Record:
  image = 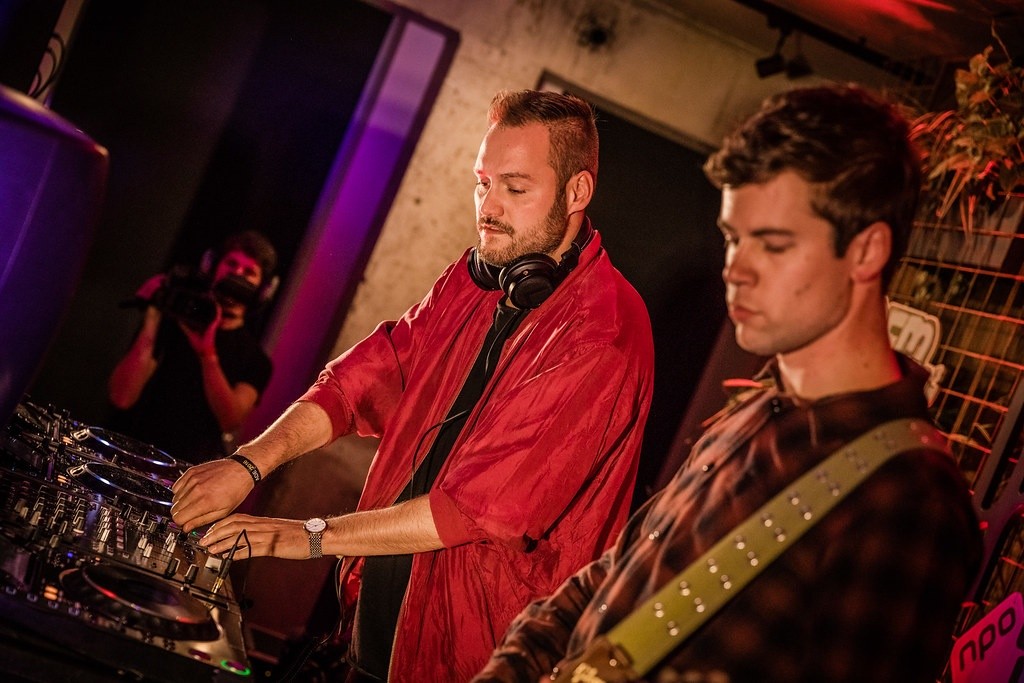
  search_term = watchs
[302,515,327,559]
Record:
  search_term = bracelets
[227,454,262,488]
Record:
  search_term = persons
[170,89,655,683]
[472,86,982,683]
[107,226,279,466]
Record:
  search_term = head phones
[198,249,279,303]
[465,214,594,312]
